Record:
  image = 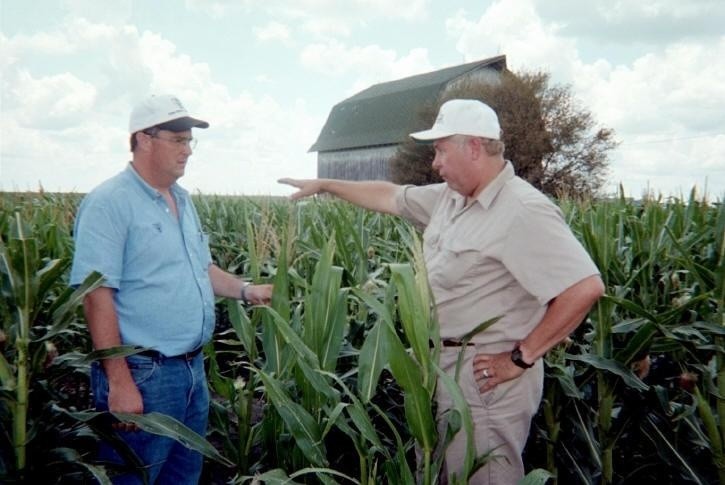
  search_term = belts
[136,345,205,363]
[424,338,476,347]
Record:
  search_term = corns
[680,371,723,453]
[0,352,16,386]
[552,336,569,374]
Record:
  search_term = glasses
[143,133,198,151]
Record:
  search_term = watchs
[510,344,535,369]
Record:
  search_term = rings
[483,369,489,378]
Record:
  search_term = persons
[67,92,275,485]
[277,98,606,485]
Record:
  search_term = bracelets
[241,282,253,305]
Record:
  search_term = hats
[128,93,209,136]
[408,98,501,144]
